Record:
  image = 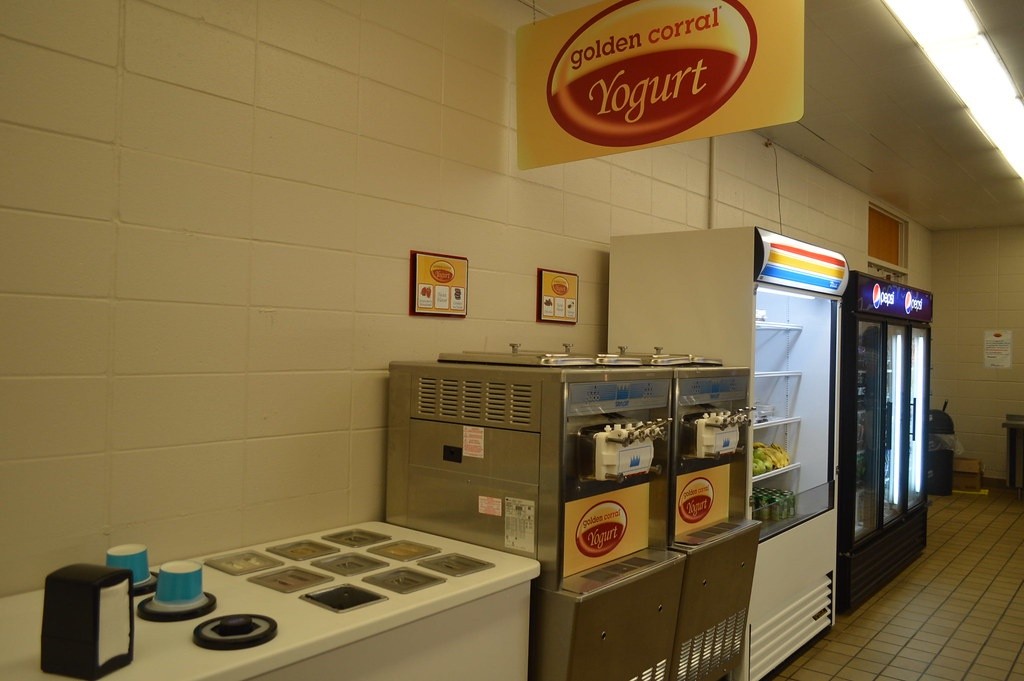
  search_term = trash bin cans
[927,409,955,496]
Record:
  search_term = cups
[153,560,205,607]
[105,544,152,586]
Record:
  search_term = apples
[753,451,772,474]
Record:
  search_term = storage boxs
[952,457,983,493]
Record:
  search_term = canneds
[753,487,794,521]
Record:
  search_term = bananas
[754,442,789,468]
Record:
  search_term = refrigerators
[841,271,933,615]
[604,225,840,681]
